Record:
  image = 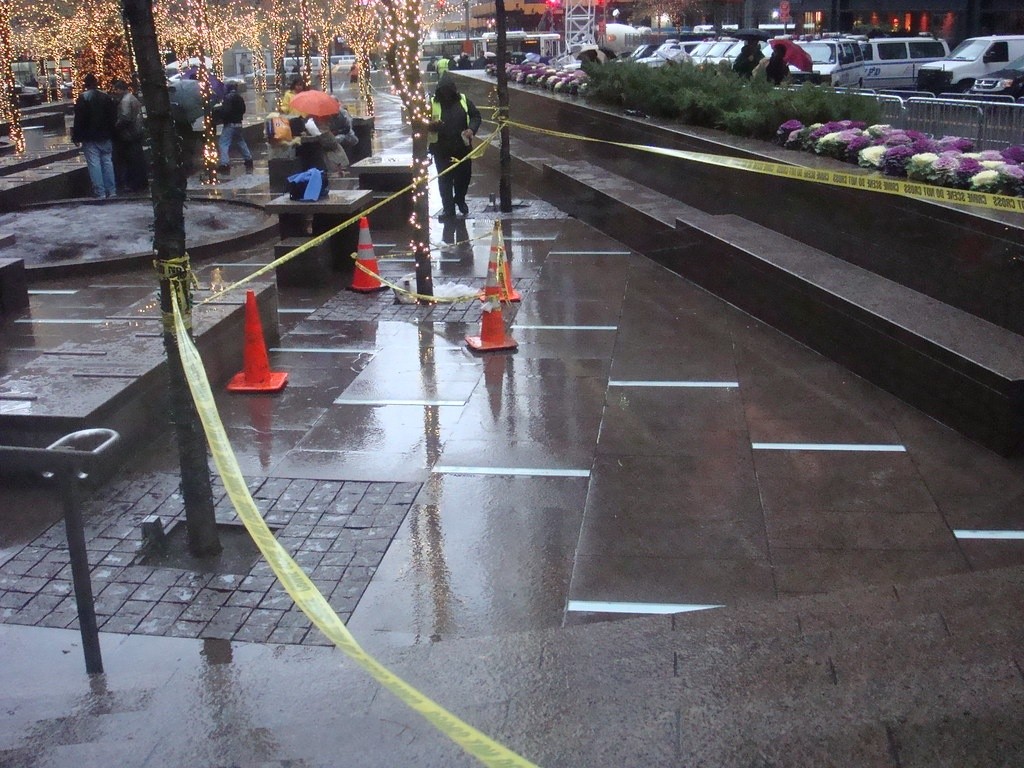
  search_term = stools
[371,192,406,229]
[274,237,332,286]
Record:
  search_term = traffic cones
[226,288,288,392]
[345,217,389,293]
[463,266,519,351]
[478,220,522,304]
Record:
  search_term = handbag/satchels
[267,116,293,142]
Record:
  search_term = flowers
[485,61,590,98]
[776,117,1024,198]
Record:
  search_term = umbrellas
[729,27,772,43]
[512,51,525,55]
[484,51,495,57]
[180,66,225,106]
[168,78,217,122]
[290,89,340,116]
[768,37,813,73]
[525,53,541,63]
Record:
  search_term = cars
[318,64,354,82]
[0,79,41,109]
[243,68,298,87]
[161,71,250,99]
[627,31,1024,99]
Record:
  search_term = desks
[265,190,373,272]
[350,156,414,213]
[351,116,375,157]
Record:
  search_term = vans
[330,54,377,72]
[282,56,334,85]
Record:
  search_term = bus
[416,31,562,69]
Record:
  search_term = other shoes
[96,192,106,199]
[438,207,455,220]
[453,195,468,213]
[244,159,254,174]
[107,191,117,197]
[217,165,230,175]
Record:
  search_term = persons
[764,43,790,86]
[350,58,359,83]
[72,73,117,199]
[214,78,253,173]
[261,79,358,159]
[733,39,765,79]
[426,51,487,79]
[425,71,482,220]
[113,76,146,191]
[506,55,523,64]
